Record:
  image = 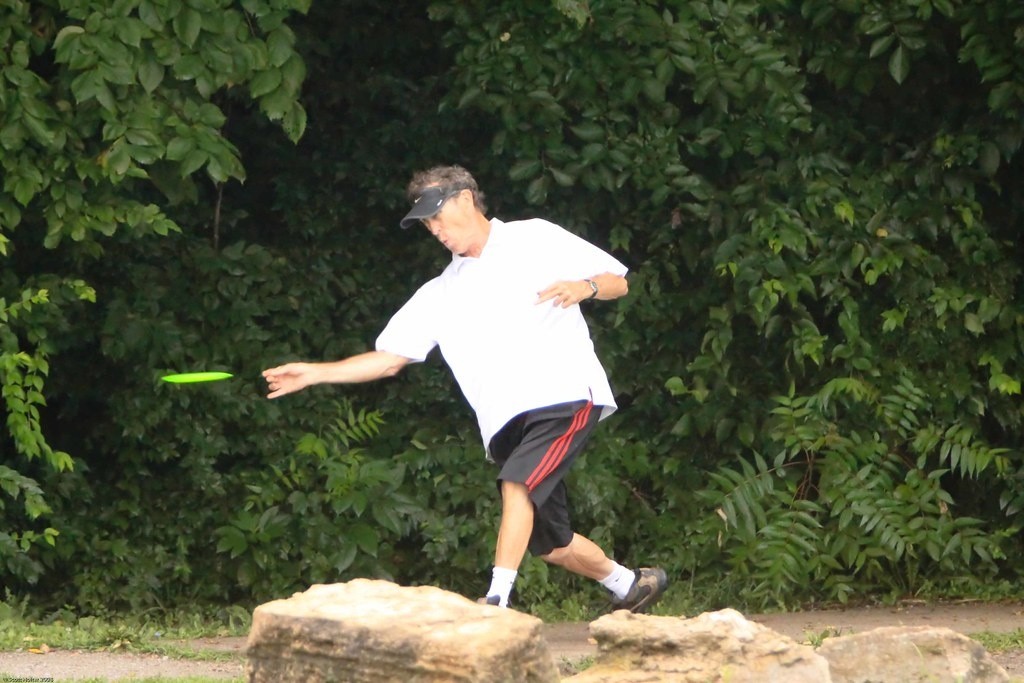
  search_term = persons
[262,166,667,613]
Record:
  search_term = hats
[400,183,470,229]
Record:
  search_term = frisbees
[159,372,234,383]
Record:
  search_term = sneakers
[478,595,511,609]
[611,567,667,614]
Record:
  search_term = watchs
[584,278,598,299]
